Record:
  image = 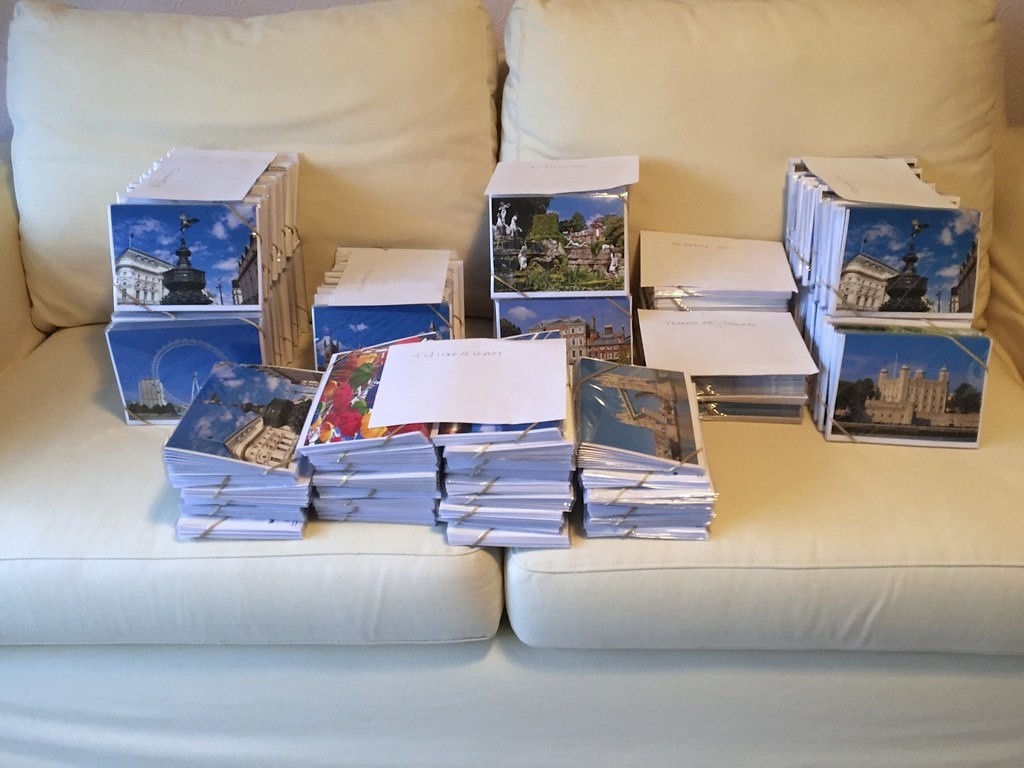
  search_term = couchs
[1,0,1024,767]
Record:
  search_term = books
[633,156,993,448]
[490,192,633,364]
[105,153,309,427]
[311,247,465,375]
[163,330,720,550]
